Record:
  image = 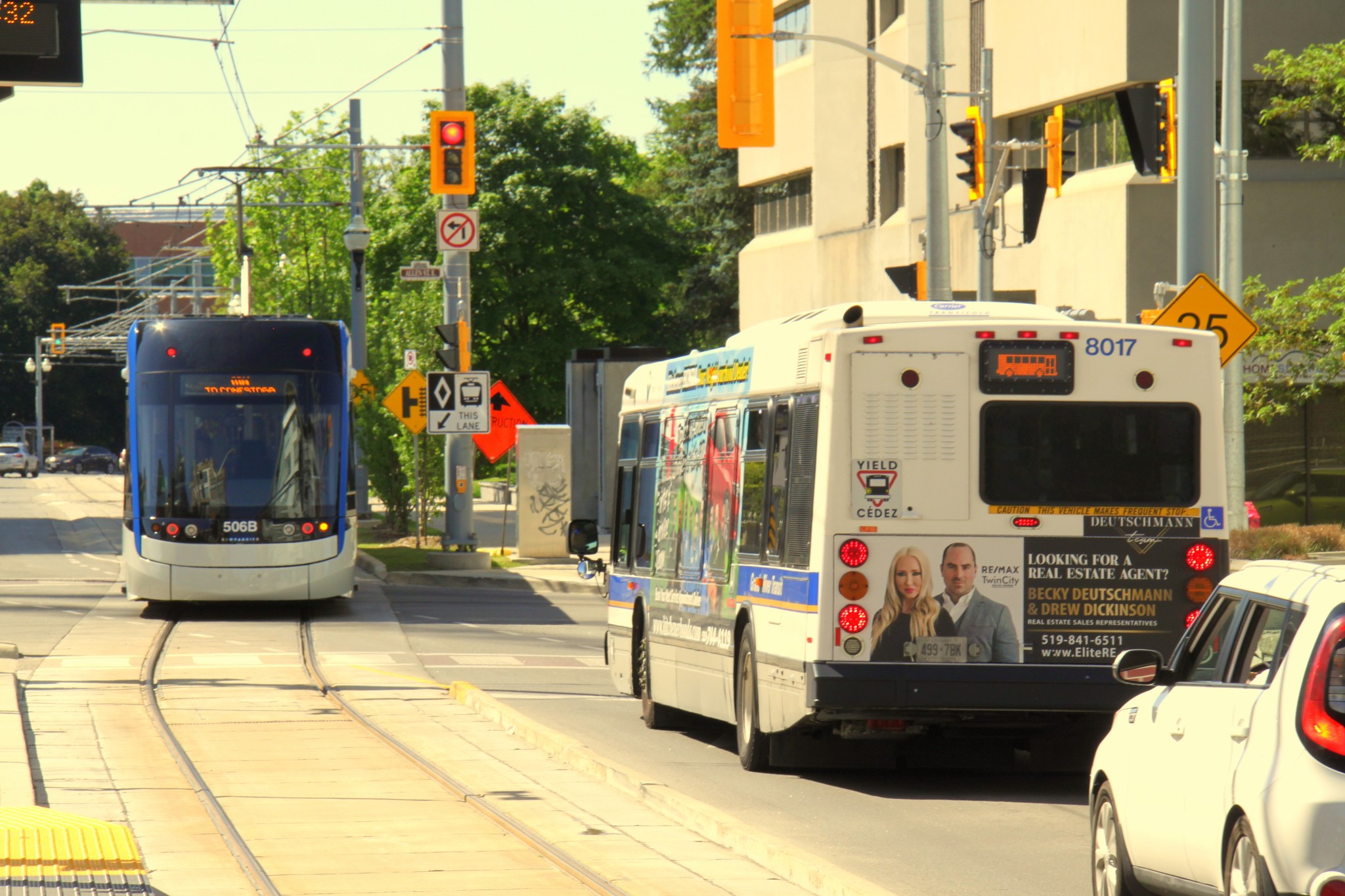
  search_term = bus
[119,319,357,609]
[568,302,1233,775]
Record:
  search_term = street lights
[26,358,53,465]
[119,366,130,476]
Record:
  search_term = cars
[0,441,40,479]
[44,444,118,475]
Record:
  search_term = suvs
[1082,552,1345,896]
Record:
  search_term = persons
[869,546,957,662]
[933,543,1019,663]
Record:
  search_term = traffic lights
[950,106,988,202]
[430,110,477,196]
[0,0,84,86]
[884,263,927,301]
[1046,104,1081,199]
[1115,79,1178,185]
[49,323,66,355]
